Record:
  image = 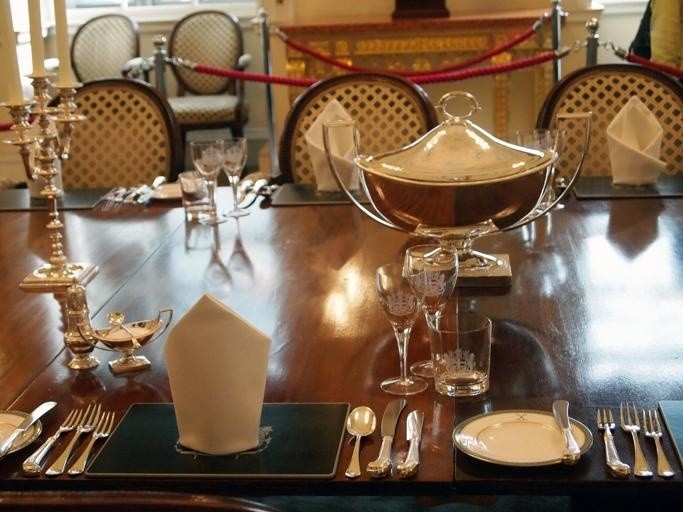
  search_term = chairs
[278,72,438,185]
[65,11,142,87]
[166,9,251,181]
[533,63,683,180]
[42,77,185,184]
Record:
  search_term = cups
[180,168,210,222]
[431,308,494,396]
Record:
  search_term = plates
[452,407,593,470]
[0,406,42,455]
[145,184,185,203]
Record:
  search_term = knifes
[366,397,408,479]
[0,400,58,458]
[396,410,423,477]
[551,397,581,463]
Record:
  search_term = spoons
[345,404,376,480]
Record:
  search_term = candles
[51,1,75,85]
[0,0,23,103]
[26,0,48,79]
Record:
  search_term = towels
[162,292,274,456]
[303,94,359,193]
[604,91,666,191]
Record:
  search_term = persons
[629,0,683,81]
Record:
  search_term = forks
[97,182,143,214]
[642,404,675,479]
[617,399,653,478]
[20,403,115,482]
[593,403,630,477]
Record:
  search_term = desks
[0,179,682,512]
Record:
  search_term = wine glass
[403,245,458,379]
[372,263,433,394]
[189,133,254,225]
[511,126,567,222]
